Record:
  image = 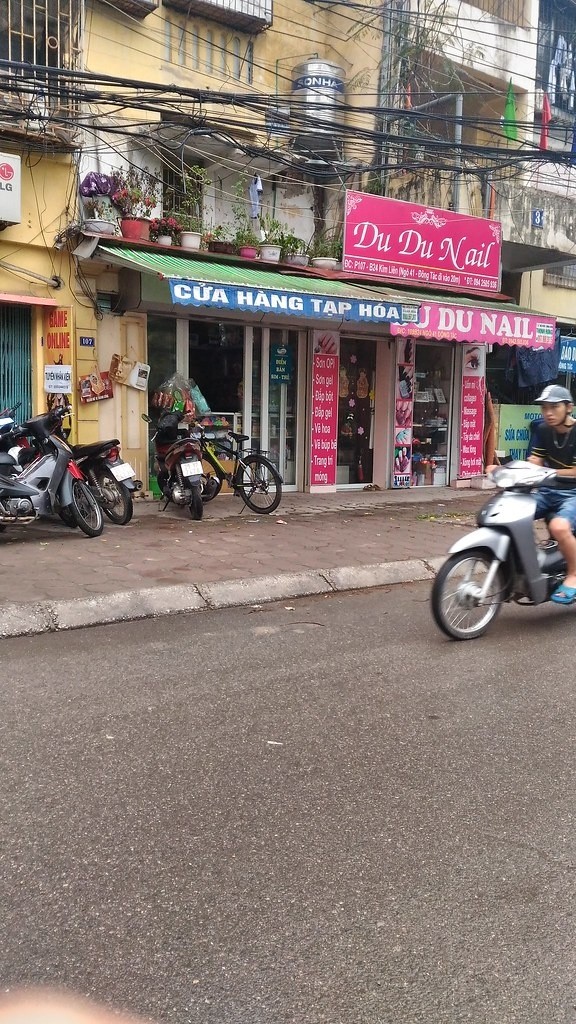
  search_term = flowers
[112,188,158,220]
[149,216,183,236]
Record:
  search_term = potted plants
[83,200,115,234]
[180,165,342,271]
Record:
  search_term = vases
[118,217,153,241]
[158,235,172,246]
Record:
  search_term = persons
[396,401,413,426]
[395,428,411,444]
[394,447,410,473]
[317,332,338,354]
[399,339,413,363]
[397,365,414,399]
[485,385,576,602]
[465,346,485,375]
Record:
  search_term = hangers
[248,166,260,187]
[560,327,576,343]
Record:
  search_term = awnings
[96,243,423,325]
[346,279,558,351]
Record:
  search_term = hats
[534,385,574,403]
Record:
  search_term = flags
[539,94,552,150]
[502,80,517,143]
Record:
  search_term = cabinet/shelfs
[411,371,449,485]
[148,412,297,492]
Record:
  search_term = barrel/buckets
[149,476,162,498]
[291,58,346,157]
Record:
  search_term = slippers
[550,584,576,604]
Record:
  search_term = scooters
[429,459,575,641]
[139,410,204,521]
[0,401,143,537]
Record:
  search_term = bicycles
[189,415,283,514]
